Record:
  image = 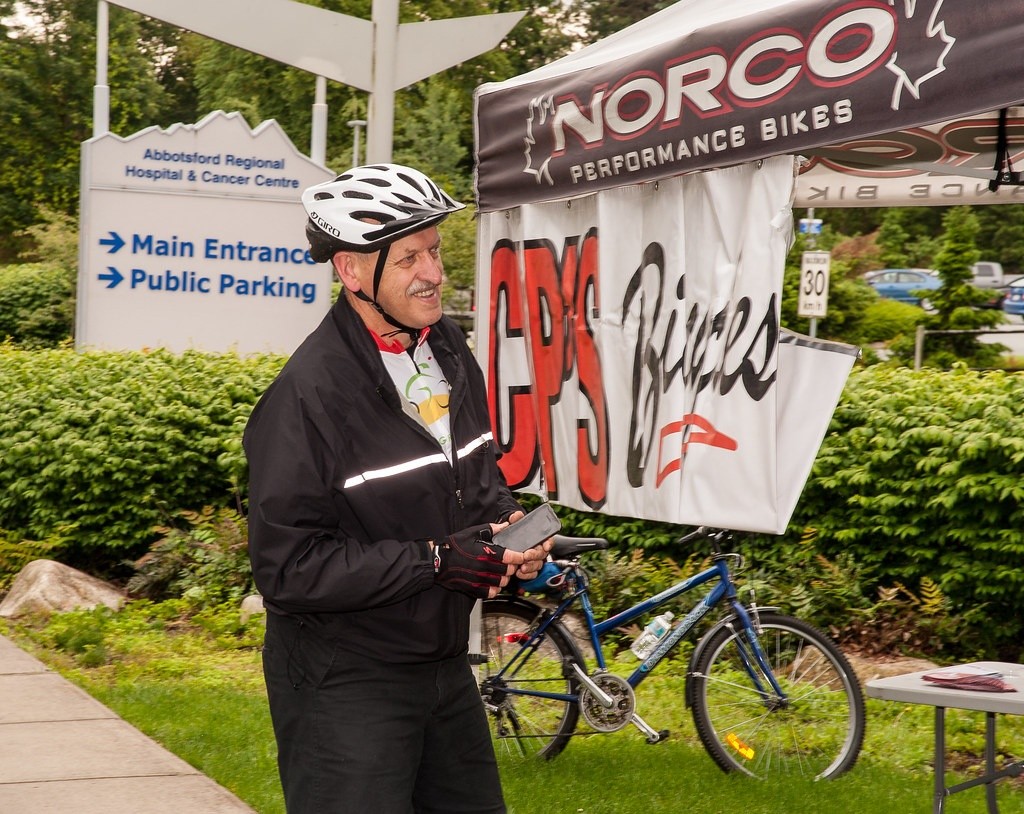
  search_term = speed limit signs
[800,248,832,319]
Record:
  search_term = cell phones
[492,503,562,553]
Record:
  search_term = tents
[469,0,1024,665]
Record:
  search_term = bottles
[629,610,675,661]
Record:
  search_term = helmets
[301,163,467,264]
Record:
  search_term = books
[920,664,1000,683]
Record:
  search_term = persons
[242,161,554,814]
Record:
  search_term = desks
[865,661,1024,814]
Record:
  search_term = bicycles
[472,516,868,794]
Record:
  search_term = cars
[856,267,946,312]
[969,263,1024,315]
